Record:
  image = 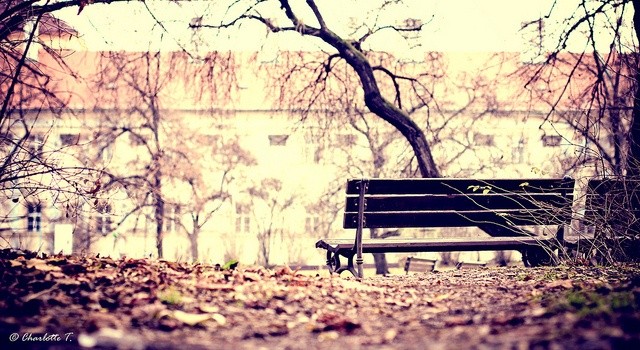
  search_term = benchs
[561,179,640,263]
[314,176,574,279]
